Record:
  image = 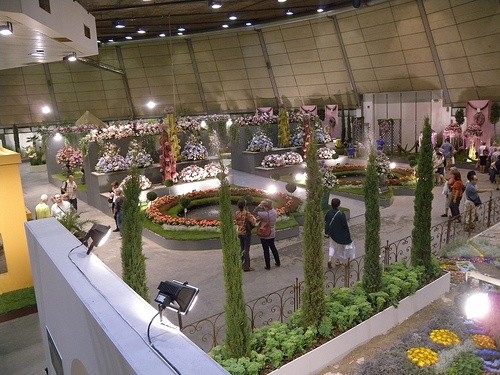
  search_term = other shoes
[328,262,332,268]
[113,228,120,232]
[441,214,448,217]
[264,266,270,271]
[275,262,280,266]
[244,267,255,272]
[335,261,343,266]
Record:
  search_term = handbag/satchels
[256,210,272,237]
[344,242,355,260]
[442,181,450,196]
[61,180,67,194]
[474,196,482,206]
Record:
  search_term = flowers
[24,106,485,244]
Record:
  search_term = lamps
[81,223,111,254]
[155,279,200,316]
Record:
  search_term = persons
[433,137,454,184]
[376,136,385,155]
[464,172,495,232]
[51,194,74,220]
[235,200,257,273]
[475,141,500,183]
[325,198,352,268]
[340,140,366,158]
[252,199,281,271]
[35,194,51,219]
[28,145,44,165]
[61,175,80,217]
[441,171,463,223]
[109,183,125,232]
